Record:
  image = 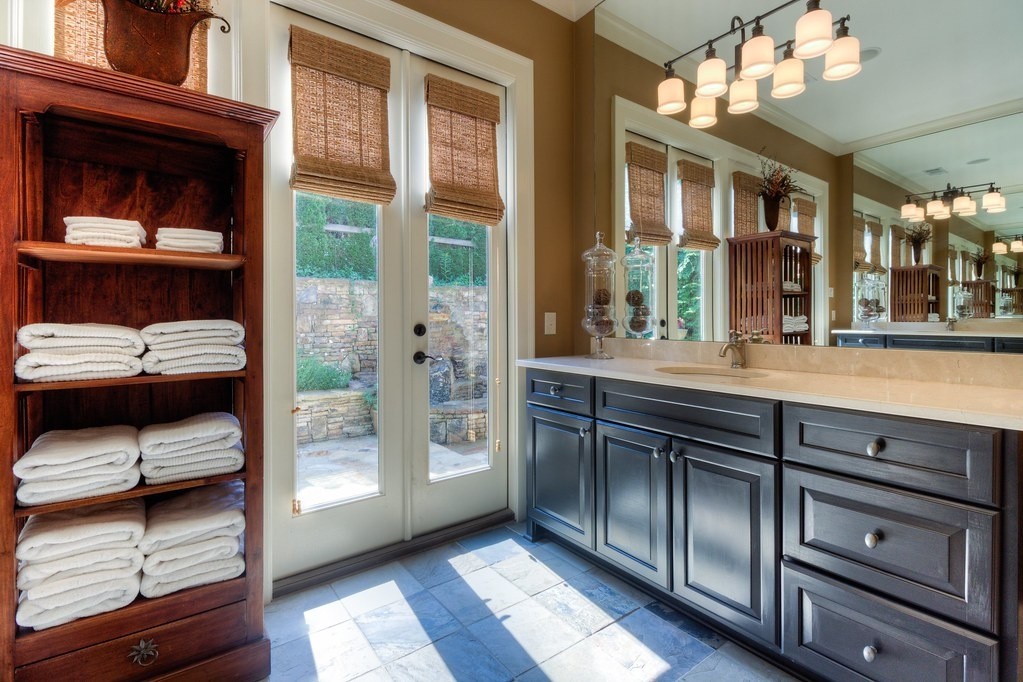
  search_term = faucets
[946,318,957,332]
[748,328,775,345]
[718,329,748,369]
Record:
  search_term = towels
[137,478,247,598]
[140,320,247,375]
[16,500,146,631]
[136,411,246,485]
[155,227,224,254]
[927,313,940,322]
[926,295,936,301]
[12,424,142,506]
[62,216,148,248]
[783,315,809,333]
[783,281,801,292]
[13,323,146,382]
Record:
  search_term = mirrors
[946,191,1022,321]
[594,0,1022,356]
[852,110,1022,322]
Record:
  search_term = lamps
[899,181,1006,223]
[992,234,1022,255]
[689,15,861,128]
[657,0,834,115]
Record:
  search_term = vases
[1015,273,1019,286]
[101,0,231,86]
[913,243,921,264]
[764,196,780,232]
[976,262,982,279]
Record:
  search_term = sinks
[652,366,771,382]
[912,328,946,334]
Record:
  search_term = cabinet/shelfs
[837,334,1023,353]
[962,281,996,319]
[725,230,819,347]
[526,367,1023,682]
[1001,288,1023,316]
[891,265,944,323]
[0,43,281,682]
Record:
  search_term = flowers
[754,146,809,212]
[129,0,216,16]
